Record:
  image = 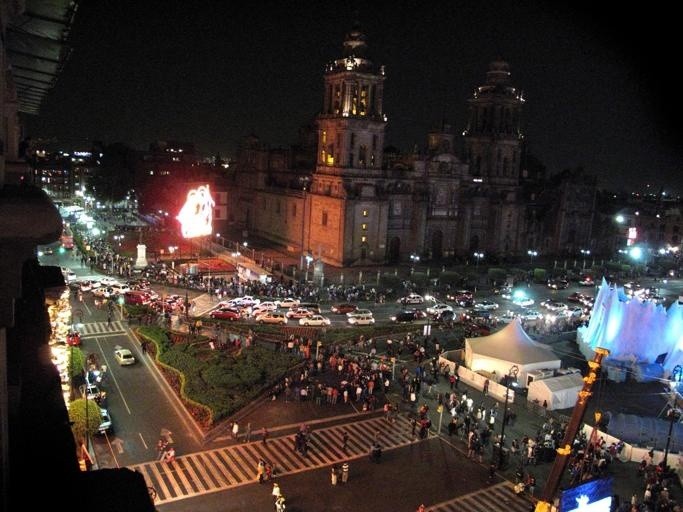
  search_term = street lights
[618,248,627,264]
[298,175,308,271]
[67,311,86,402]
[579,250,591,269]
[408,254,419,281]
[493,365,519,468]
[663,366,682,468]
[526,250,537,266]
[84,354,105,454]
[471,252,483,291]
[303,254,313,282]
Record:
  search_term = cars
[347,315,375,325]
[211,294,321,325]
[79,384,113,437]
[59,266,193,315]
[345,308,372,319]
[546,279,569,290]
[624,280,664,305]
[298,314,331,325]
[44,248,52,255]
[579,277,595,287]
[113,348,134,366]
[395,287,596,325]
[330,303,359,315]
[66,329,79,345]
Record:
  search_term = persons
[62,238,683,512]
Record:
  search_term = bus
[62,230,73,249]
